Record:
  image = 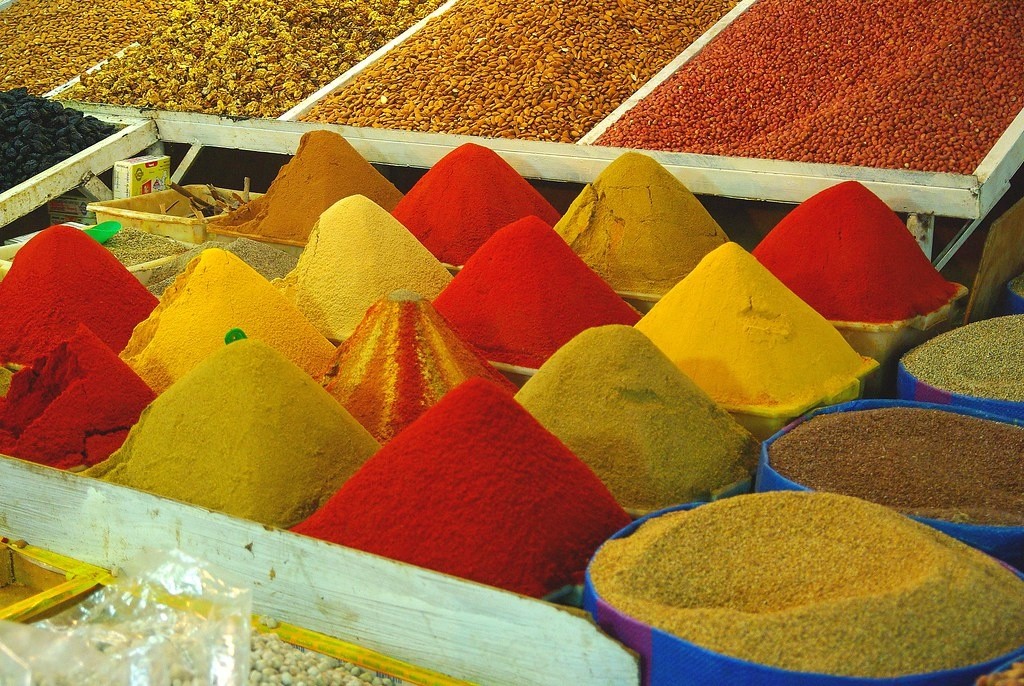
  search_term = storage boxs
[111,154,171,200]
[0,184,971,604]
[49,212,97,226]
[48,192,95,216]
